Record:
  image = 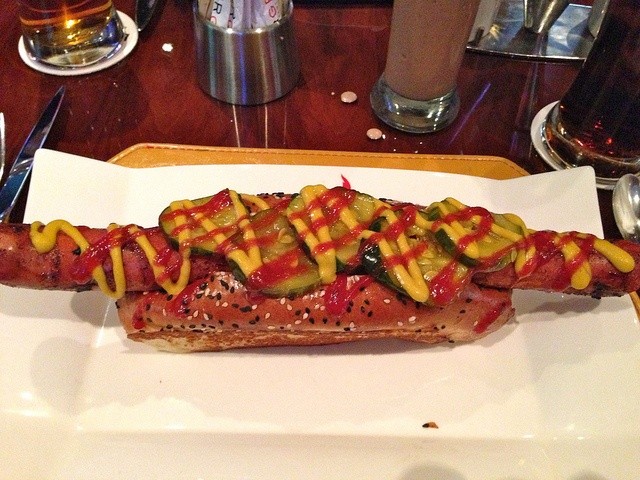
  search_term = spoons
[611,172,640,240]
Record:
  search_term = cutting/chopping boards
[101,139,533,180]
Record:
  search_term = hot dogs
[1,184,640,350]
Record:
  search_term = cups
[20,0,125,67]
[368,1,482,136]
[541,0,639,184]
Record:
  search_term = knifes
[0,82,68,229]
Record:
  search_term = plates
[17,8,139,78]
[0,148,640,437]
[529,98,626,192]
